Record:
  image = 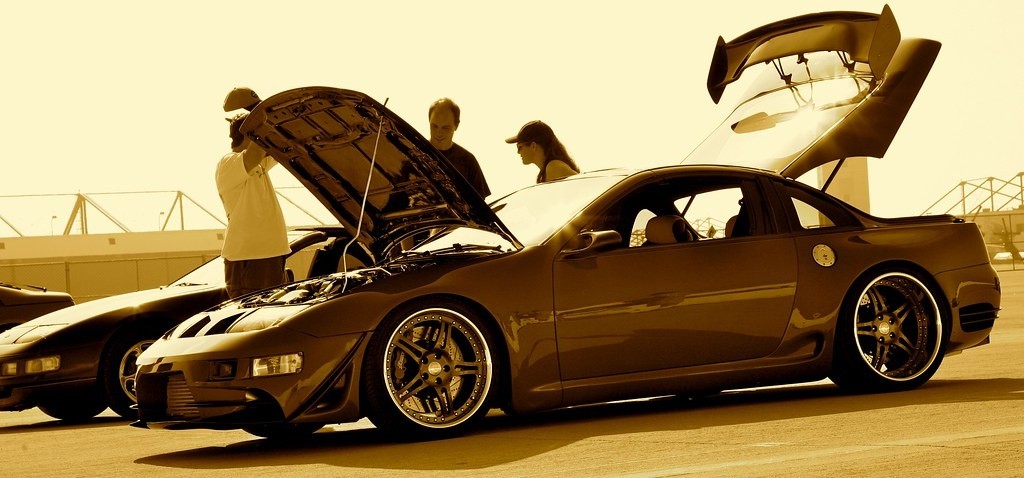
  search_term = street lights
[159,211,165,232]
[51,215,56,236]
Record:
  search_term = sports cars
[124,4,1001,442]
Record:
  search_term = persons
[215,87,292,300]
[505,121,580,184]
[428,98,491,201]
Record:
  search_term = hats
[223,87,262,111]
[505,120,554,144]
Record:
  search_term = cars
[0,224,374,425]
[0,281,76,336]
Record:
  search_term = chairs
[641,215,691,248]
[724,213,748,236]
